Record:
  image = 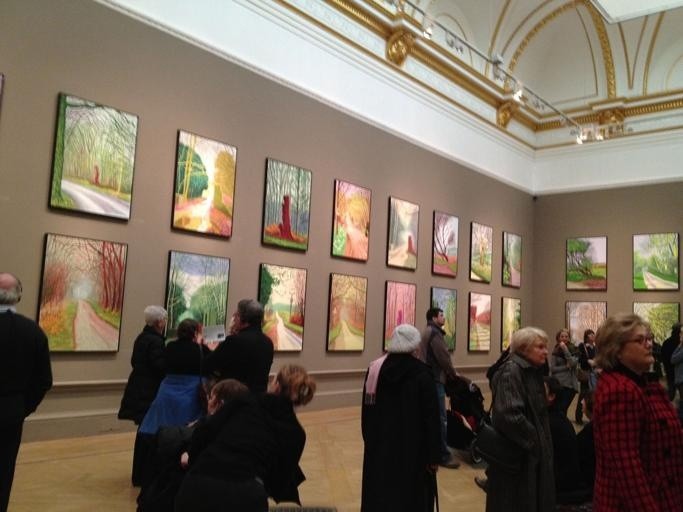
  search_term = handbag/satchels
[576,369,590,383]
[474,421,506,464]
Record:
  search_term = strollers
[446,376,493,466]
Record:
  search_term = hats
[384,324,422,353]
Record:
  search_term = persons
[118,301,315,510]
[358,310,683,511]
[1,271,54,512]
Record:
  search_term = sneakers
[475,475,488,493]
[442,456,460,468]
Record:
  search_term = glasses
[623,334,651,344]
